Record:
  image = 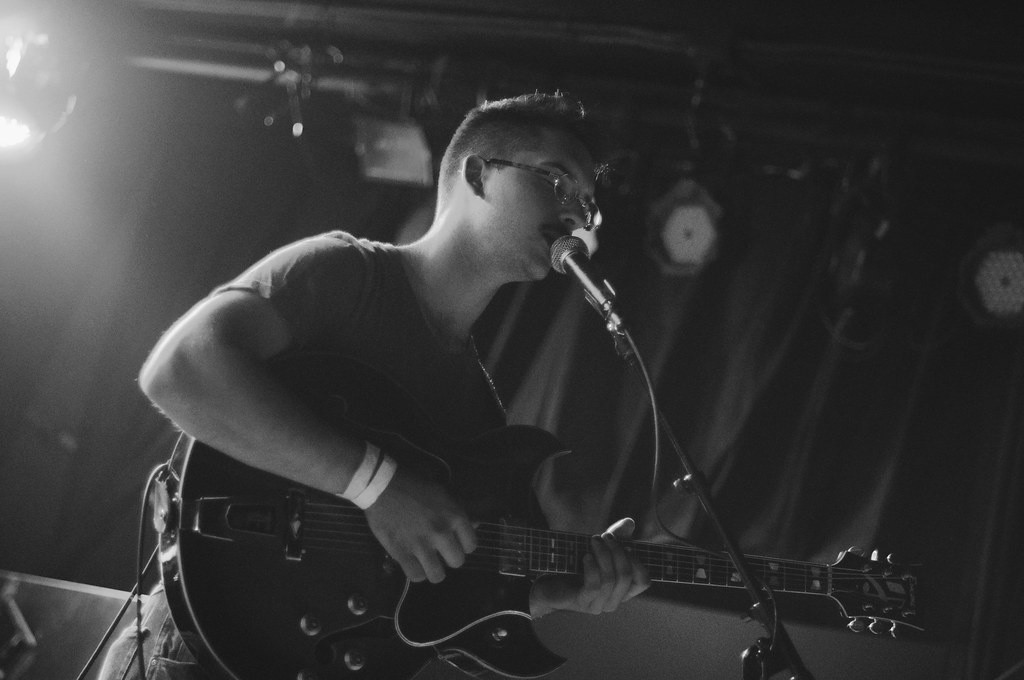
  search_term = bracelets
[337,440,399,510]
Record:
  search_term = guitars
[151,348,929,680]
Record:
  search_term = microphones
[549,235,629,332]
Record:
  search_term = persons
[96,89,652,680]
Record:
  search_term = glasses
[486,158,602,232]
